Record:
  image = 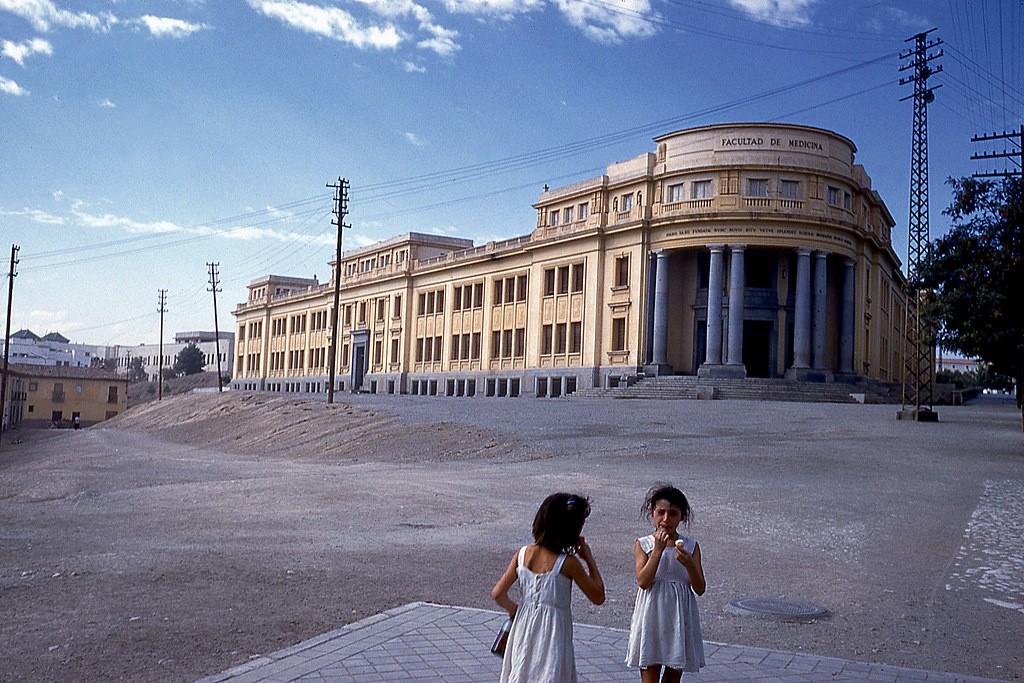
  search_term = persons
[74,416,80,430]
[492,492,605,683]
[623,481,706,683]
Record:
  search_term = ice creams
[675,539,684,552]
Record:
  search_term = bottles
[490,615,514,656]
